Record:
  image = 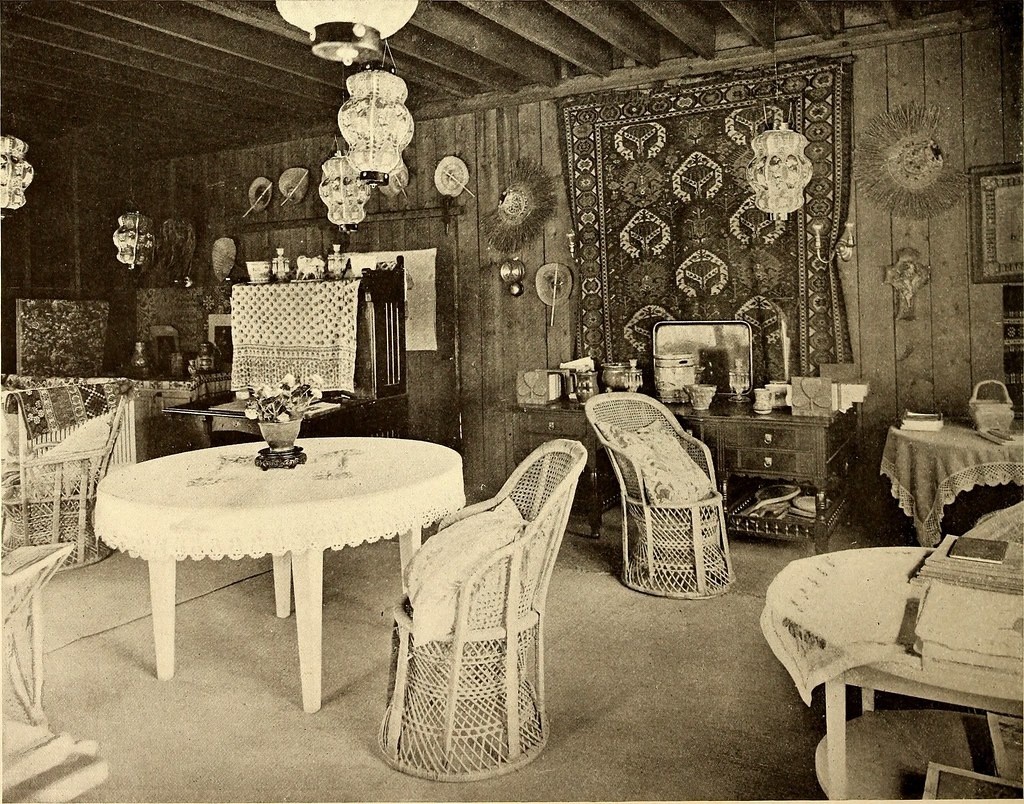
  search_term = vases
[258,419,303,451]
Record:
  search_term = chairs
[585,392,736,599]
[377,438,588,781]
[1,395,127,570]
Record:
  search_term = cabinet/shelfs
[517,399,858,556]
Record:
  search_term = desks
[94,438,466,713]
[759,546,1024,801]
[162,392,409,453]
[880,420,1024,547]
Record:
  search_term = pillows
[49,418,110,452]
[593,419,712,506]
[403,497,530,648]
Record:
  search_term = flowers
[244,372,325,423]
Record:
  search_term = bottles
[130,342,150,367]
[576,370,600,409]
[188,359,196,374]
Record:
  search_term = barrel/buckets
[653,351,695,402]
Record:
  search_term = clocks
[500,259,524,297]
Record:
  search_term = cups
[682,384,717,411]
[170,352,183,376]
[765,384,788,408]
[245,261,271,284]
[752,388,774,415]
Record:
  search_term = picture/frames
[969,161,1024,284]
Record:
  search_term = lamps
[813,220,856,263]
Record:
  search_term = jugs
[194,339,222,373]
